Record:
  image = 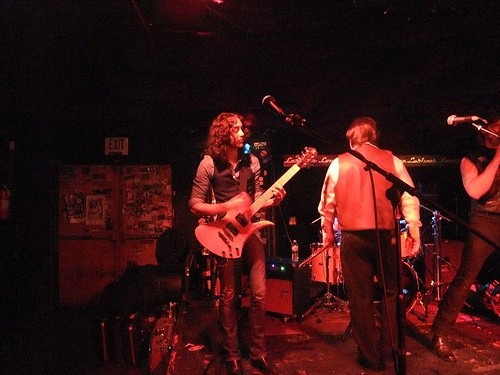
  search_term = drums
[370,261,421,319]
[307,241,343,286]
[397,222,425,259]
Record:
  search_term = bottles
[0,185,11,219]
[292,240,299,262]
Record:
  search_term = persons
[432,114,500,363]
[318,117,423,373]
[190,113,286,375]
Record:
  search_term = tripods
[418,202,470,309]
[295,239,348,322]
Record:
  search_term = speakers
[237,274,311,318]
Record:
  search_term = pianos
[283,153,444,169]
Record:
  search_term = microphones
[261,95,285,116]
[446,115,481,126]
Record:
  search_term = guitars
[192,145,317,259]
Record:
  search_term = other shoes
[228,359,244,375]
[357,356,385,372]
[251,356,279,375]
[429,335,457,363]
